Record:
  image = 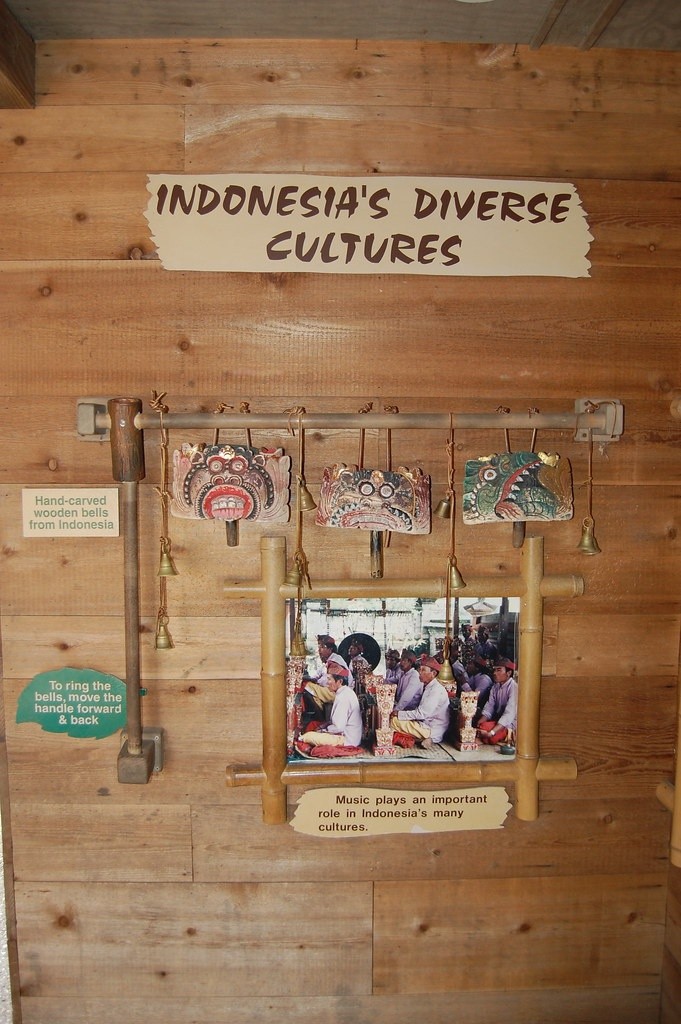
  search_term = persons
[294,633,374,754]
[453,623,518,746]
[379,651,453,749]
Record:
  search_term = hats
[493,658,515,670]
[351,640,364,653]
[385,649,401,662]
[421,654,442,671]
[326,660,348,677]
[401,648,418,664]
[468,651,488,667]
[318,634,335,644]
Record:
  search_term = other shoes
[417,737,434,749]
